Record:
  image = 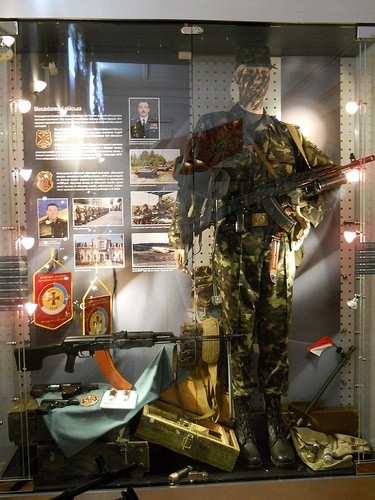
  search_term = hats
[234,43,278,70]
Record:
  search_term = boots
[264,395,296,467]
[233,395,263,470]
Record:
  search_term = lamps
[346,294,360,309]
[344,230,360,244]
[12,167,33,182]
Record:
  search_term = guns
[31,383,99,398]
[36,398,80,415]
[177,154,375,244]
[14,330,244,391]
[49,463,139,500]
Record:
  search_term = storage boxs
[281,406,359,437]
[134,400,240,472]
[7,393,149,478]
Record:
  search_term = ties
[142,119,146,128]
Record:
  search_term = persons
[168,45,339,469]
[133,199,170,224]
[130,101,159,138]
[39,204,68,238]
[74,204,111,225]
